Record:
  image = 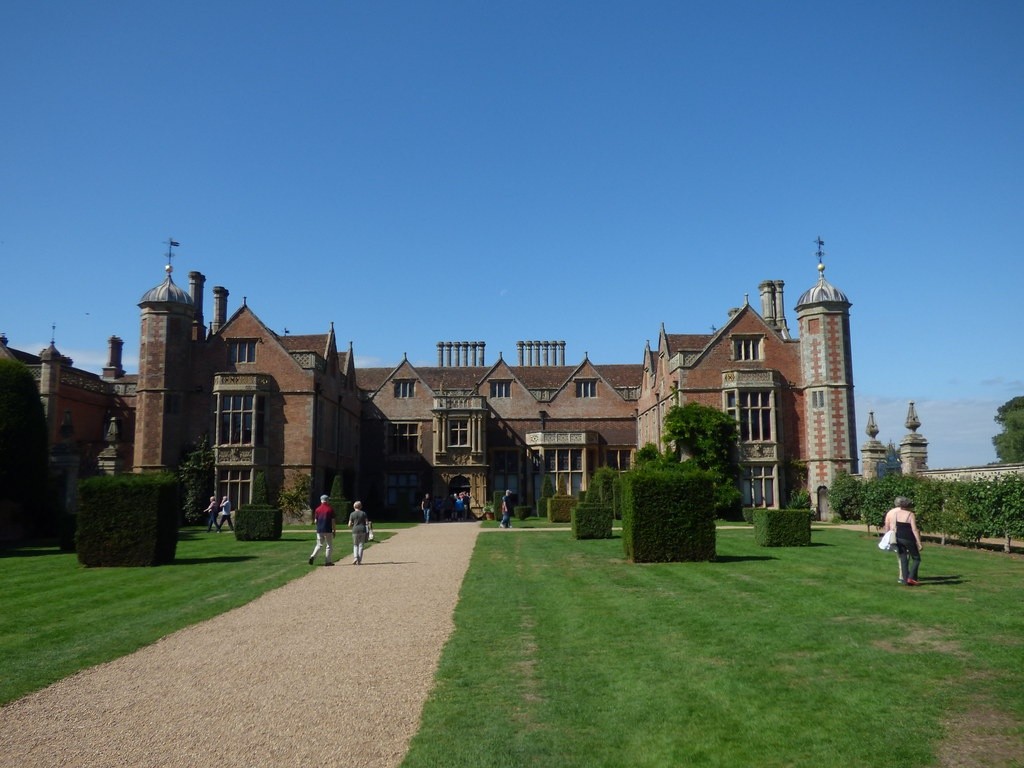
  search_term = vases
[418,511,424,520]
[485,511,493,520]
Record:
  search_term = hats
[320,495,331,500]
[506,490,512,493]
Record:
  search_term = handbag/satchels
[889,526,898,545]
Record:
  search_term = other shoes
[217,529,221,533]
[232,529,234,531]
[907,578,919,585]
[309,558,313,565]
[426,520,429,523]
[507,525,512,528]
[500,524,504,528]
[353,558,358,565]
[898,579,905,584]
[216,529,218,532]
[358,561,361,564]
[325,563,335,566]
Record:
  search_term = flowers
[483,504,494,513]
[414,498,433,511]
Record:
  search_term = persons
[500,490,513,528]
[422,491,471,524]
[308,495,336,566]
[216,496,234,532]
[203,497,221,533]
[348,501,369,565]
[878,497,923,587]
